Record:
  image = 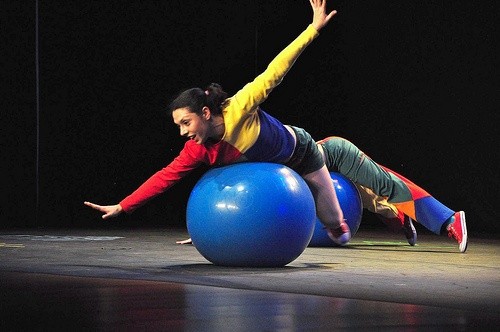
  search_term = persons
[84,0,351,244]
[316,136,468,253]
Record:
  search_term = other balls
[187,162,316,266]
[308,171,363,247]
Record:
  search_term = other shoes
[324,220,351,244]
[448,210,469,253]
[403,215,417,245]
